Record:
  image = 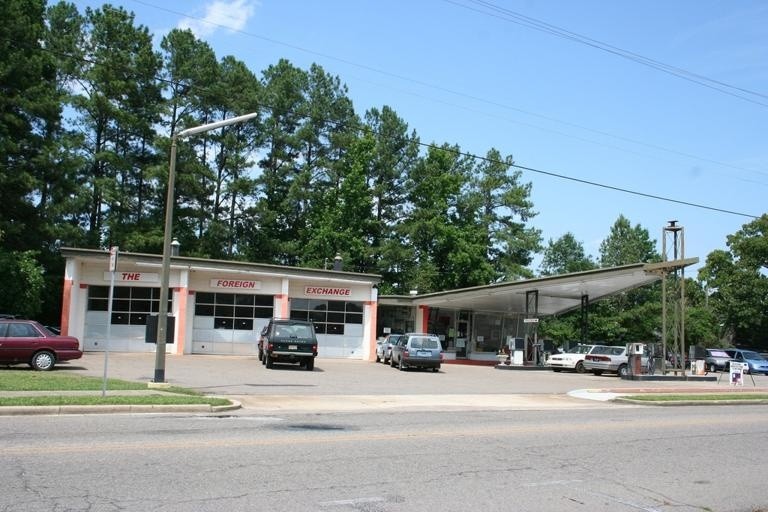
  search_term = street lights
[154,111,258,384]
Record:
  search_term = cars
[545,344,595,373]
[668,352,690,369]
[585,346,656,377]
[0,312,28,322]
[695,348,735,373]
[258,326,299,365]
[374,333,405,363]
[543,342,558,355]
[725,350,767,375]
[500,336,542,364]
[0,318,83,369]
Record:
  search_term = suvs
[262,316,318,370]
[390,332,445,372]
